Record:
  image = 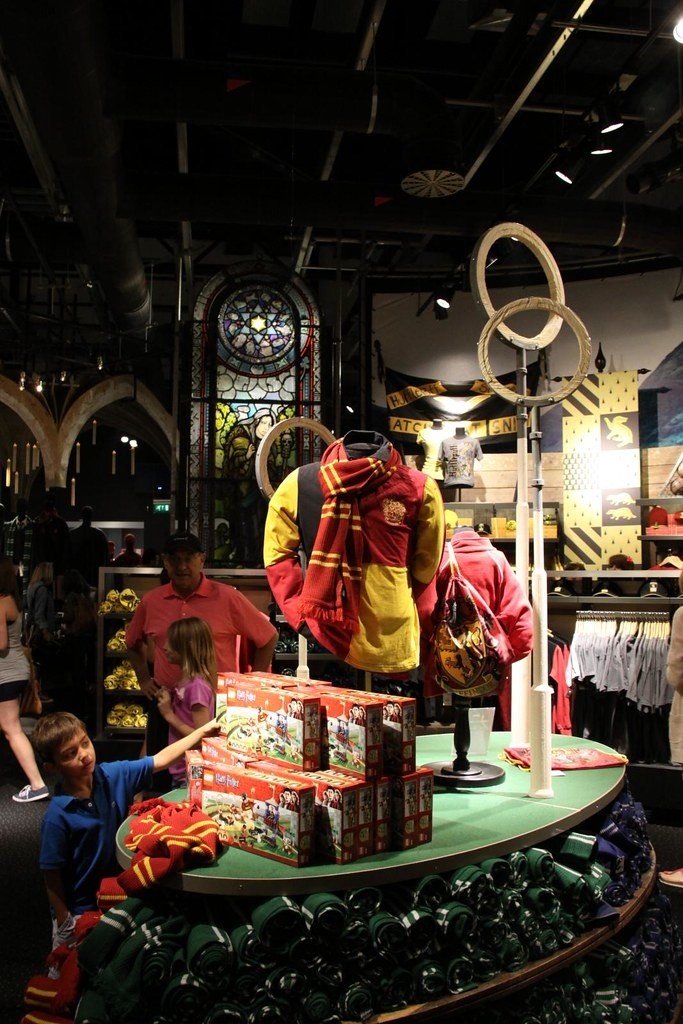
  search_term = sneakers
[12,782,49,802]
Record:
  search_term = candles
[5,420,135,505]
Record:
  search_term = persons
[219,697,432,856]
[109,534,167,592]
[21,562,56,704]
[554,555,636,591]
[667,571,683,767]
[154,617,217,789]
[125,531,280,804]
[33,711,220,979]
[416,419,483,487]
[0,498,110,590]
[58,571,97,694]
[0,555,49,803]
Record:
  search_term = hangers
[572,609,672,648]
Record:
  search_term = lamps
[435,284,457,309]
[595,103,624,134]
[589,121,613,155]
[554,157,586,185]
[19,355,103,393]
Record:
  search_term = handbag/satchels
[430,575,514,696]
[20,620,42,649]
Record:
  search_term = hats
[157,533,203,559]
[549,577,682,597]
[659,555,683,569]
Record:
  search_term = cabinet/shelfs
[96,568,341,734]
[636,497,683,540]
[442,501,560,543]
[511,570,683,605]
[29,730,656,1024]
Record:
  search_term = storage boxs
[183,669,435,869]
